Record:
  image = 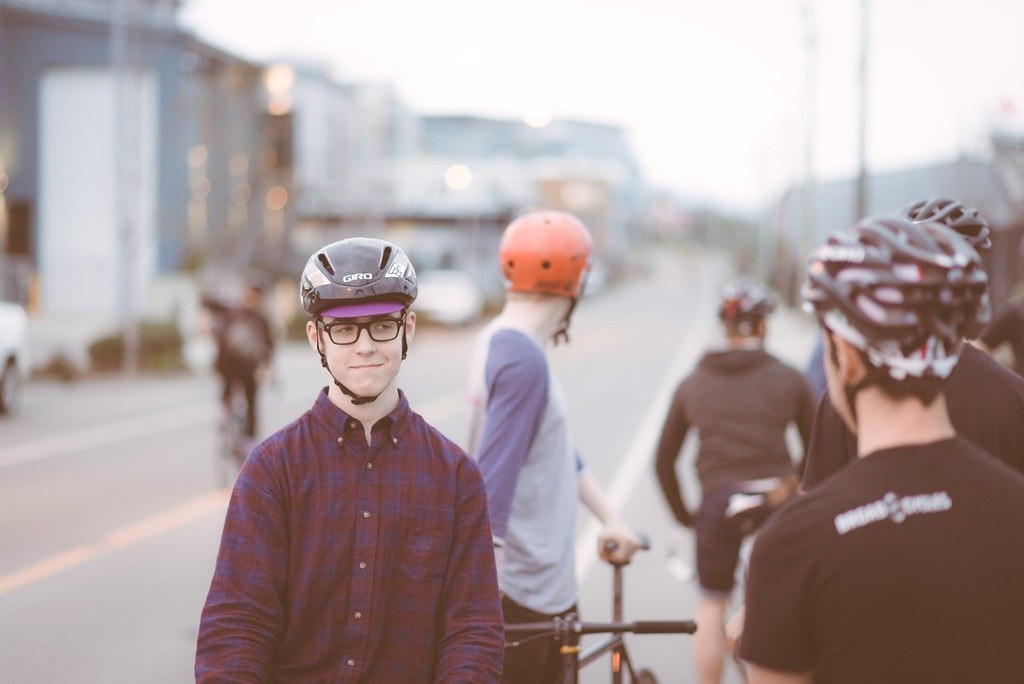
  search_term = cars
[412,266,485,329]
[0,297,34,419]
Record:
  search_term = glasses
[315,309,405,344]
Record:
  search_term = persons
[194,237,504,684]
[202,278,275,462]
[459,208,639,684]
[797,192,1022,485]
[652,283,816,680]
[729,214,1023,684]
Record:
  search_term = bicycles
[501,532,697,684]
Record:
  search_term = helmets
[300,236,418,319]
[898,198,991,251]
[717,288,780,322]
[800,211,992,374]
[500,212,593,297]
